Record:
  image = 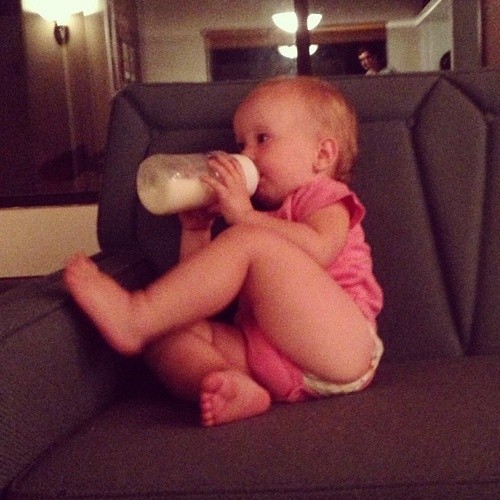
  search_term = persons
[65,75,384,426]
[356,44,397,75]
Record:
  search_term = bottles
[137,154,260,215]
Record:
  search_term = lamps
[270,11,322,58]
[21,1,106,177]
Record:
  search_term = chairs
[0,68,500,496]
